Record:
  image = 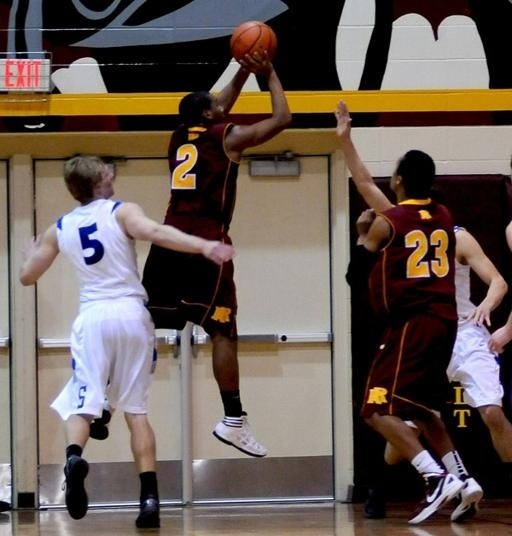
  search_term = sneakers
[88,386,114,440]
[135,496,159,529]
[212,414,268,457]
[64,454,89,520]
[362,485,386,519]
[450,476,484,522]
[407,468,463,526]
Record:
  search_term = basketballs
[230,21,277,65]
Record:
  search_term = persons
[331,220,512,519]
[88,42,293,459]
[488,153,512,356]
[332,102,486,525]
[19,152,235,528]
